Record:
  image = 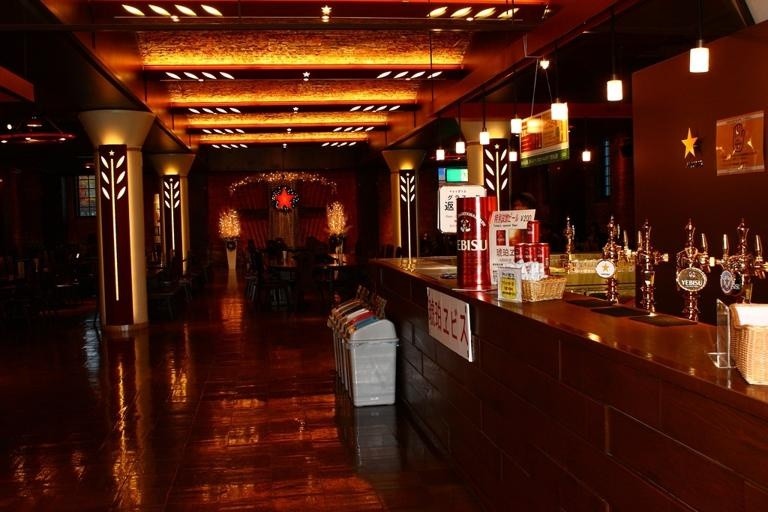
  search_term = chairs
[243,240,401,317]
[150,255,181,320]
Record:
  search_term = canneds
[513,220,551,278]
[496,228,506,246]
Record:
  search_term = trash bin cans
[328,298,399,408]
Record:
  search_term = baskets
[523,276,567,303]
[729,302,768,387]
